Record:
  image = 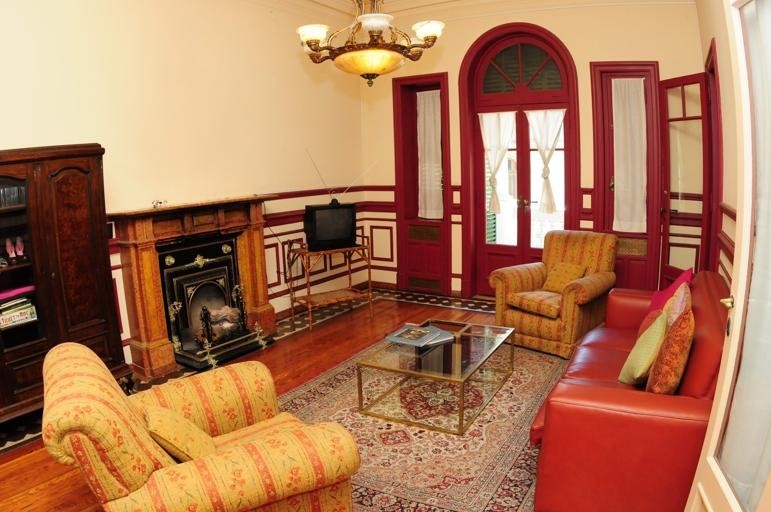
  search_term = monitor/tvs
[303,204,357,252]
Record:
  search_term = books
[385,323,441,348]
[424,326,455,345]
[0,297,33,315]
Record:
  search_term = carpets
[275,336,570,512]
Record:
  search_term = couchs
[529,271,731,512]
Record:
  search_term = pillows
[144,404,217,462]
[645,305,694,394]
[637,309,661,338]
[543,263,587,293]
[662,282,692,333]
[643,267,692,311]
[619,311,669,383]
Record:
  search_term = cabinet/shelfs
[284,234,374,330]
[0,142,136,448]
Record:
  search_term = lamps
[295,0,445,86]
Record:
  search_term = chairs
[41,341,361,512]
[489,229,618,359]
